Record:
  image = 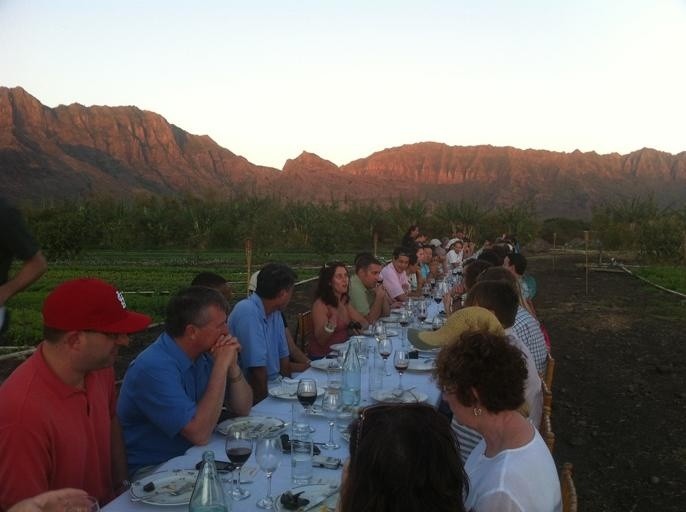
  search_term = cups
[290,442,314,484]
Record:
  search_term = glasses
[348,402,441,484]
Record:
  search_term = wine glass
[227,425,251,500]
[292,271,461,450]
[253,432,278,510]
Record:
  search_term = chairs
[539,405,555,451]
[560,462,578,512]
[545,352,555,389]
[538,368,553,406]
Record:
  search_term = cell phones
[312,455,341,470]
[196,461,236,472]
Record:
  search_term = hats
[41,276,153,335]
[248,269,262,293]
[405,305,505,352]
[429,238,443,248]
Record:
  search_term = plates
[268,384,325,402]
[216,415,286,441]
[274,483,342,511]
[133,468,202,507]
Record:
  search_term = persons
[0,195,48,327]
[0,277,153,512]
[116,284,255,484]
[7,486,92,512]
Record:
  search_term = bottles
[190,452,232,509]
